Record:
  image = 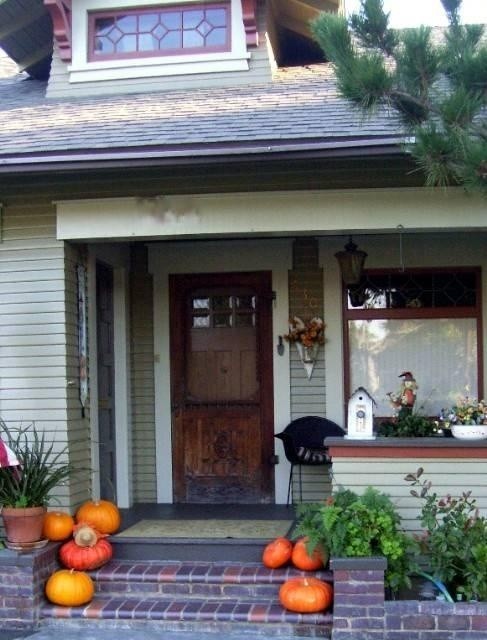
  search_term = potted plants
[0,413,122,551]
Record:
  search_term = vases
[450,423,487,441]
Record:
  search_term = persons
[394,371,418,419]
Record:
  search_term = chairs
[271,413,346,513]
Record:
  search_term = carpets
[114,517,294,541]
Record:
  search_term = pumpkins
[263,537,326,570]
[279,576,331,614]
[44,499,121,569]
[45,567,93,606]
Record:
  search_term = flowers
[281,311,327,366]
[438,380,486,429]
[374,376,442,440]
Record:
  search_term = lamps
[332,234,368,288]
